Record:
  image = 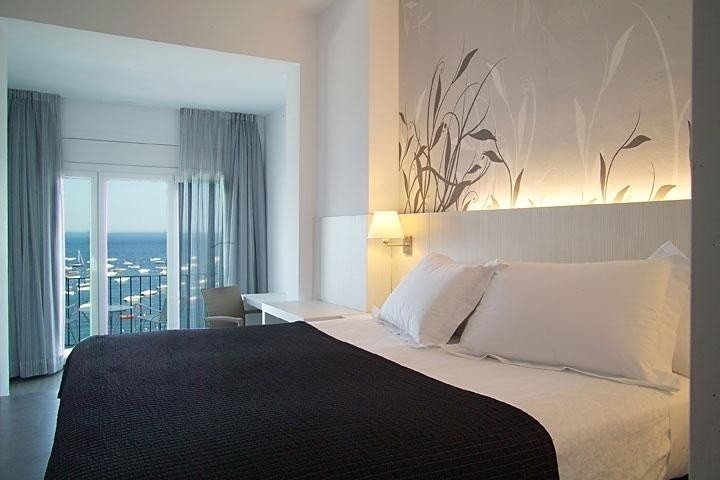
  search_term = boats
[65,247,222,321]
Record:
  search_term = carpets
[0,394,60,479]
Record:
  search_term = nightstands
[241,289,367,324]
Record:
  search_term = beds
[43,201,691,480]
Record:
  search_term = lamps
[369,211,414,256]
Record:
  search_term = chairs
[64,296,188,345]
[201,285,263,327]
